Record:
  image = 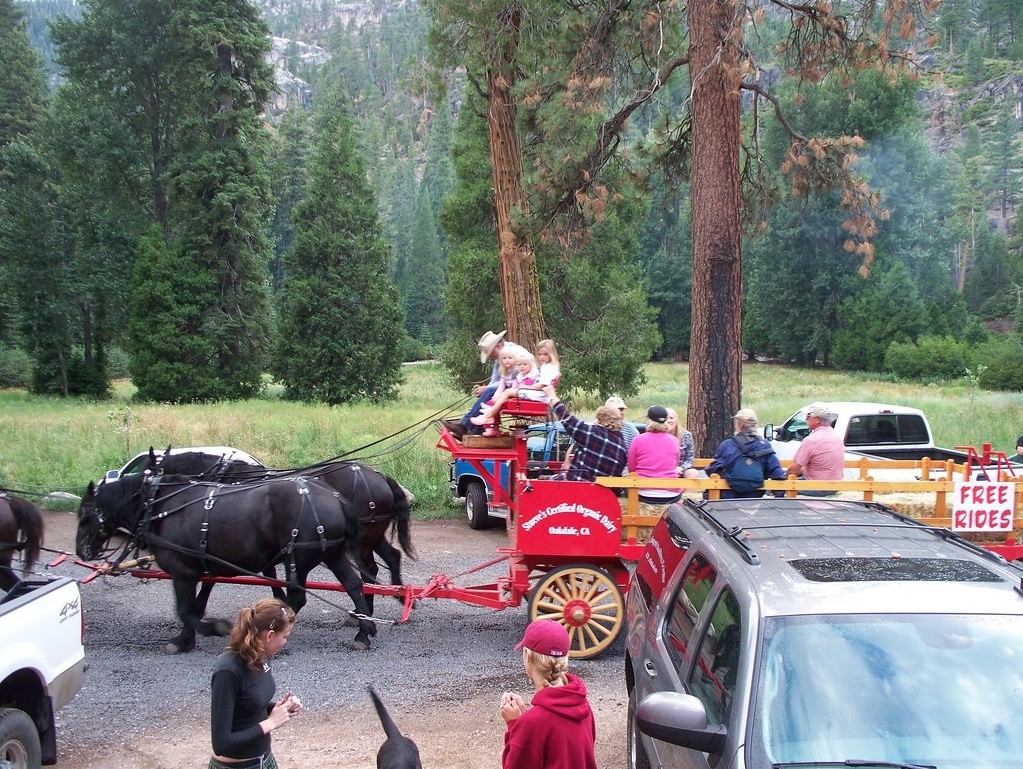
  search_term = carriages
[74,410,1022,660]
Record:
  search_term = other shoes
[442,419,468,435]
[470,414,496,425]
[482,429,500,436]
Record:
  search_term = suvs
[624,493,1021,769]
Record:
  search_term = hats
[646,405,667,423]
[514,619,570,657]
[478,329,508,364]
[731,409,757,424]
[801,401,829,417]
[605,397,627,409]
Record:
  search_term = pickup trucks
[1,575,90,769]
[449,419,647,531]
[734,400,1022,505]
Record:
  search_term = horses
[75,473,379,655]
[0,484,45,594]
[118,444,421,629]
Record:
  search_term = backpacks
[724,436,775,493]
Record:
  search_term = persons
[537,383,698,506]
[686,407,788,499]
[439,328,561,442]
[209,598,303,768]
[499,619,600,768]
[765,401,844,499]
